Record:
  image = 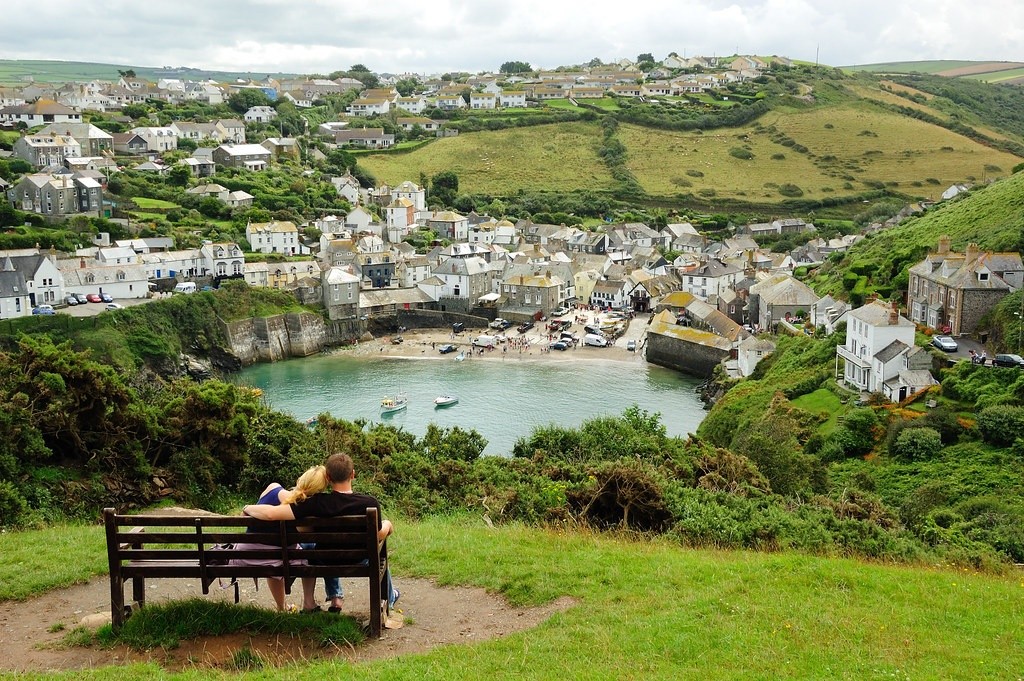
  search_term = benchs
[103,506,392,638]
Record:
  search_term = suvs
[992,354,1024,371]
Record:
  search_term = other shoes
[391,590,400,604]
[299,604,325,614]
[328,606,342,615]
[283,604,295,613]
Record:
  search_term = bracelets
[243,505,248,512]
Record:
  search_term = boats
[434,401,458,411]
[434,393,457,405]
[380,393,408,413]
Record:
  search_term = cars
[439,345,453,354]
[32,305,54,315]
[627,340,636,351]
[548,318,608,351]
[490,318,510,331]
[931,335,958,352]
[105,303,125,311]
[64,293,114,306]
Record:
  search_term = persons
[970,349,987,365]
[432,342,436,351]
[450,330,530,358]
[229,451,400,615]
[537,304,616,354]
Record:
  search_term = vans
[472,335,496,348]
[173,281,196,295]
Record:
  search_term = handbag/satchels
[207,541,232,565]
